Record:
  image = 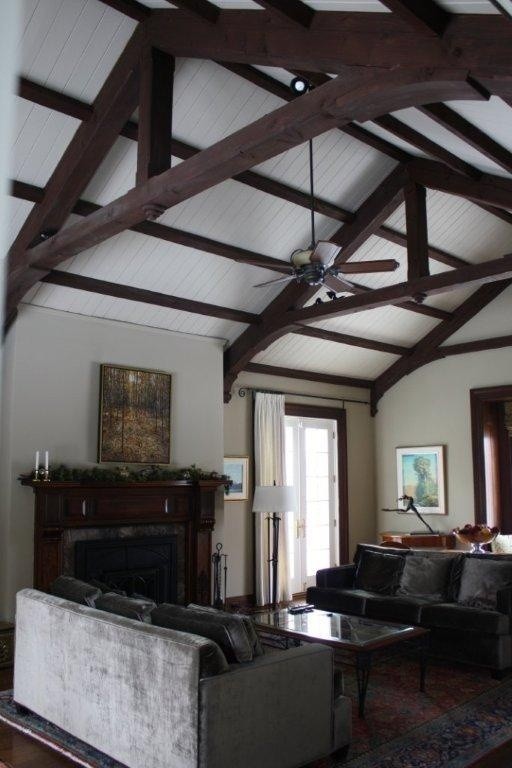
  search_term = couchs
[12,586,352,768]
[306,543,512,681]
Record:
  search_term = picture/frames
[224,455,249,501]
[396,445,447,515]
[97,364,173,468]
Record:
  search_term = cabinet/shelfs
[378,531,456,549]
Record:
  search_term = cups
[33,450,50,482]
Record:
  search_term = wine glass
[453,531,499,554]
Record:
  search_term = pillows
[448,553,512,615]
[52,575,101,608]
[401,550,462,602]
[188,603,266,658]
[353,543,410,577]
[457,558,512,611]
[149,603,256,664]
[395,555,454,604]
[351,550,405,596]
[94,591,158,624]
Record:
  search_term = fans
[253,137,400,293]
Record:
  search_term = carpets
[0,630,512,768]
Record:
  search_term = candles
[45,451,49,470]
[36,451,39,470]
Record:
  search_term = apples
[458,524,499,535]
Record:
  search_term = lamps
[252,480,297,612]
